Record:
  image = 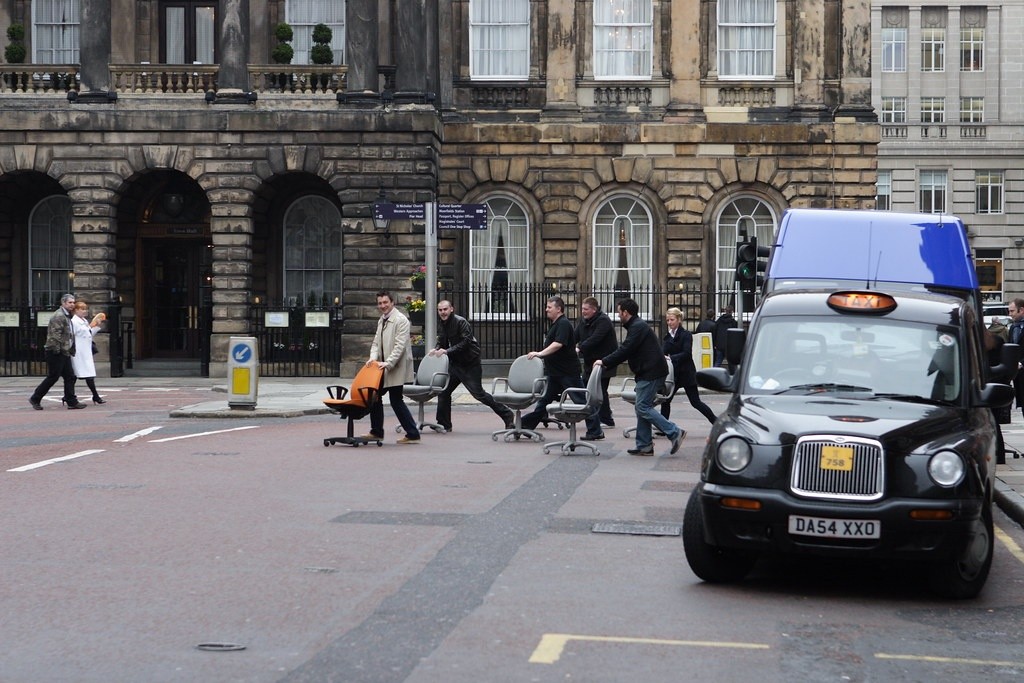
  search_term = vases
[412,346,424,356]
[412,279,425,290]
[408,312,424,325]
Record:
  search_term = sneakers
[627,447,654,456]
[670,429,688,455]
[29,398,43,410]
[360,433,384,441]
[580,432,604,440]
[397,437,421,444]
[68,402,87,409]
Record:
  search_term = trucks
[726,207,1020,382]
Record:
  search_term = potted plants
[269,25,293,86]
[4,24,28,91]
[271,291,335,361]
[311,24,332,88]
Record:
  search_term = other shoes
[430,425,452,432]
[655,432,666,436]
[600,423,615,429]
[505,413,514,429]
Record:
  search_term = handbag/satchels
[92,340,99,355]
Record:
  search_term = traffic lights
[755,236,771,286]
[735,236,757,292]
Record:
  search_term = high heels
[62,397,67,406]
[92,397,107,404]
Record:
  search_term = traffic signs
[437,203,487,229]
[374,203,425,219]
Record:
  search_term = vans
[681,282,1014,601]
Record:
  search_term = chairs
[542,366,604,455]
[394,351,450,432]
[323,361,383,446]
[489,354,549,443]
[622,356,675,437]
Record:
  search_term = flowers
[403,295,427,312]
[410,335,426,347]
[409,266,426,280]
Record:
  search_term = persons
[28,293,88,410]
[654,307,718,436]
[510,296,606,441]
[428,299,515,432]
[1003,297,1024,419]
[988,316,1009,343]
[61,301,108,406]
[695,305,738,376]
[574,296,619,429]
[592,298,689,457]
[359,289,422,444]
